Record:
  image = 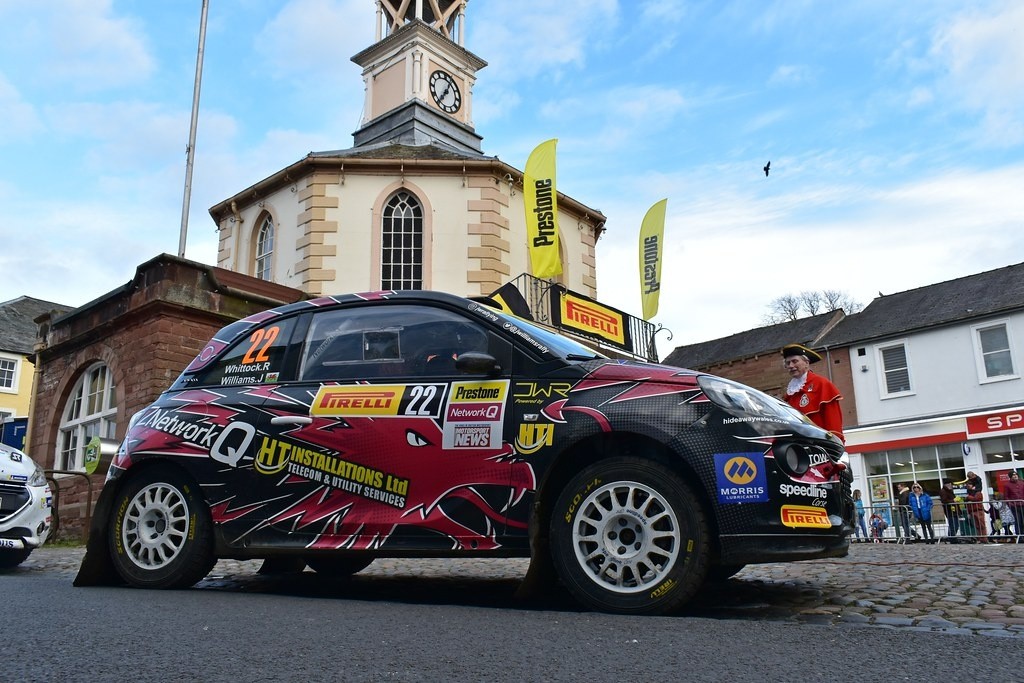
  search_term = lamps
[589,221,607,234]
[495,172,515,187]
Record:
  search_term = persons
[940,471,1024,545]
[852,489,869,542]
[909,483,935,545]
[781,344,845,446]
[897,482,921,541]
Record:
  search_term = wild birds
[764,161,771,177]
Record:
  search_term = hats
[1008,471,1018,478]
[783,344,822,364]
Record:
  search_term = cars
[70,289,858,619]
[1,445,55,565]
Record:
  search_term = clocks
[428,67,464,115]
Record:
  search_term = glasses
[914,487,919,488]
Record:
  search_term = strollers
[867,510,887,543]
